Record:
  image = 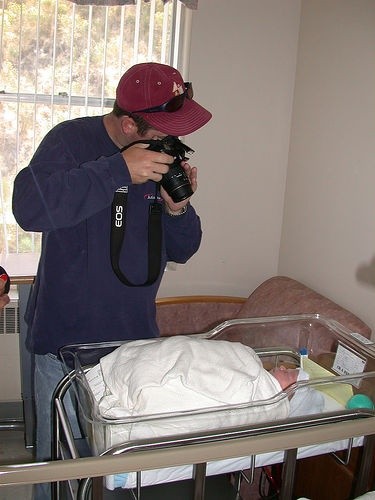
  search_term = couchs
[154,275,372,362]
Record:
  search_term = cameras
[146,135,193,203]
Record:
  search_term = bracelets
[164,202,188,217]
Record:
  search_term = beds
[50,313,375,500]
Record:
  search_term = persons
[12,62,213,500]
[124,366,310,403]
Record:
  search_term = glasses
[129,82,194,118]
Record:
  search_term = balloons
[345,394,374,412]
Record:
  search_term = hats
[289,369,310,416]
[116,63,212,137]
